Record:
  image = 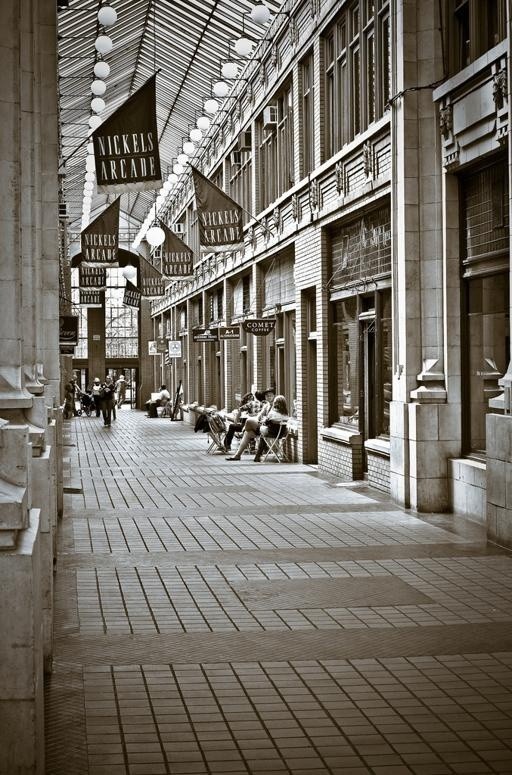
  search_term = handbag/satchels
[209,412,226,433]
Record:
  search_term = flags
[192,168,244,251]
[90,72,163,195]
[122,280,142,309]
[78,267,106,308]
[80,198,121,269]
[139,254,166,296]
[159,222,194,281]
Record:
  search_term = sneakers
[225,457,240,460]
[253,453,261,461]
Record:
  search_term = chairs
[202,408,228,456]
[158,397,173,418]
[258,419,292,463]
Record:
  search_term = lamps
[190,109,230,142]
[196,96,238,130]
[183,123,221,154]
[56,4,117,231]
[251,4,270,25]
[235,9,290,56]
[204,77,249,113]
[131,156,201,250]
[175,145,206,165]
[122,256,137,280]
[146,190,166,246]
[213,57,261,97]
[221,37,275,79]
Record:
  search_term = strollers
[77,389,96,417]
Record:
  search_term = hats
[94,378,101,383]
[119,375,124,378]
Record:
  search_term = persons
[144,384,172,419]
[214,386,289,462]
[62,372,132,428]
[144,386,162,417]
